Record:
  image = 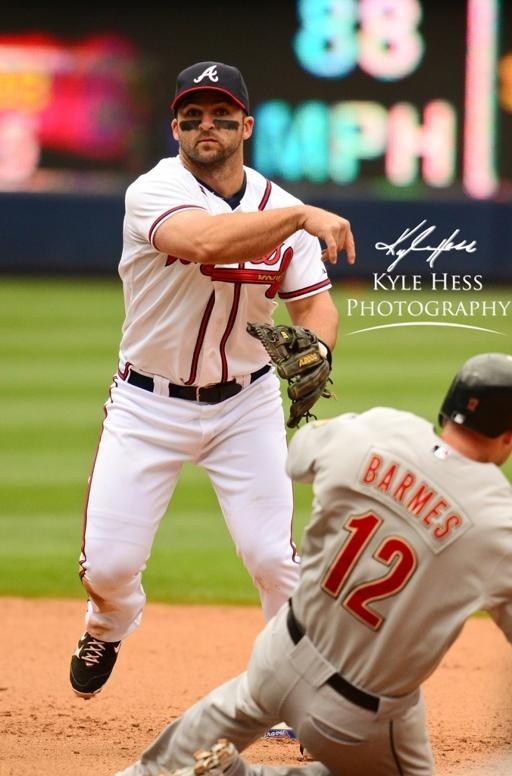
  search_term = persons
[112,353,511,776]
[69,61,357,700]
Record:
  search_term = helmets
[439,354,512,438]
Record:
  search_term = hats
[170,61,248,115]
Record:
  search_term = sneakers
[69,632,120,699]
[160,738,239,776]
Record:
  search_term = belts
[288,598,379,713]
[128,360,273,404]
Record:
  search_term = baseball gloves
[245,320,334,428]
